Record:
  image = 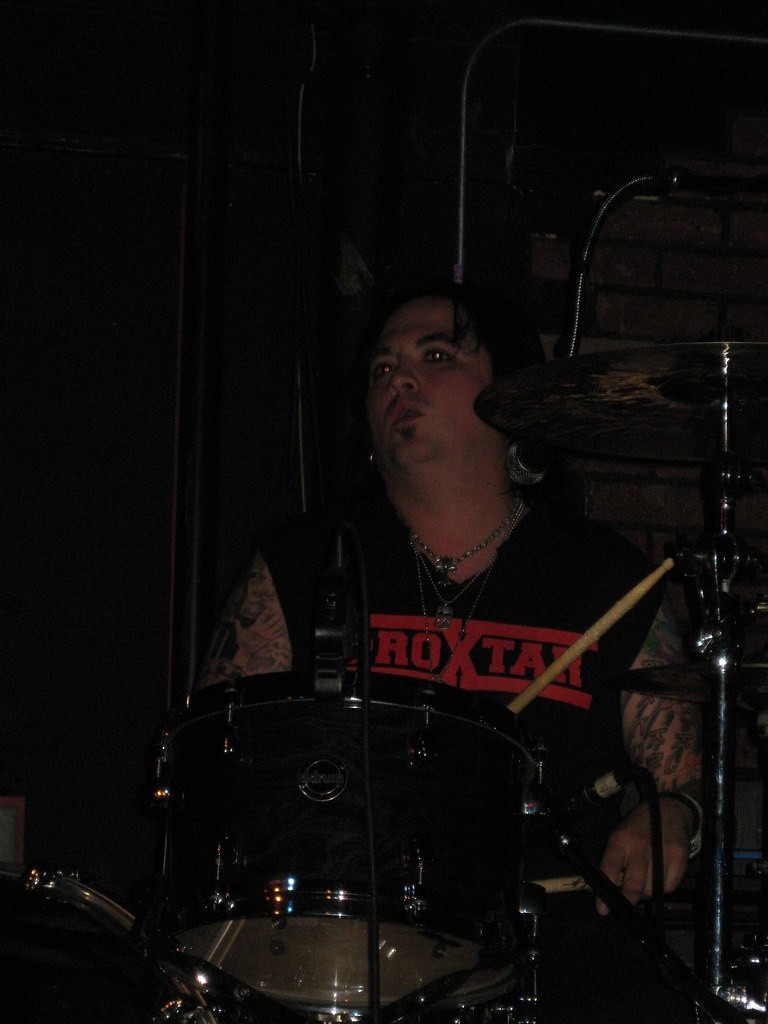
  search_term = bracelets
[660,790,709,860]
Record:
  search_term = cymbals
[469,339,768,469]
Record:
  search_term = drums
[3,860,156,1024]
[126,684,536,1018]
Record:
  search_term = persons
[181,276,711,916]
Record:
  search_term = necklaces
[393,489,531,686]
